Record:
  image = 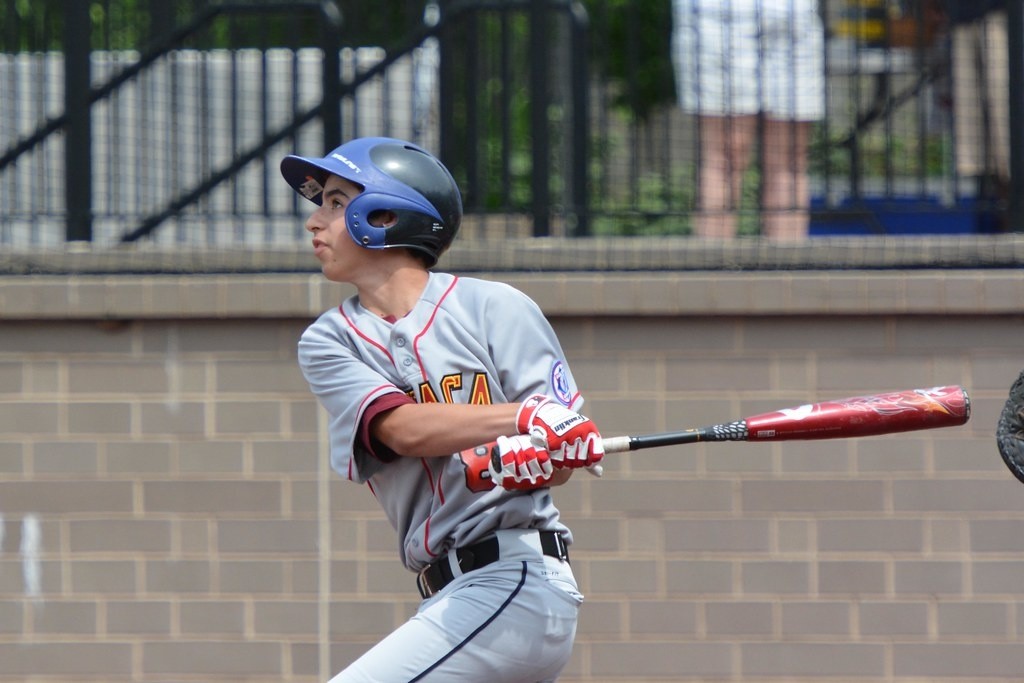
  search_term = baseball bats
[490,383,973,474]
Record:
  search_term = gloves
[488,434,553,491]
[516,394,605,476]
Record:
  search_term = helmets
[282,137,462,268]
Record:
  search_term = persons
[669,0,824,237]
[279,137,606,683]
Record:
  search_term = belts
[421,531,569,598]
